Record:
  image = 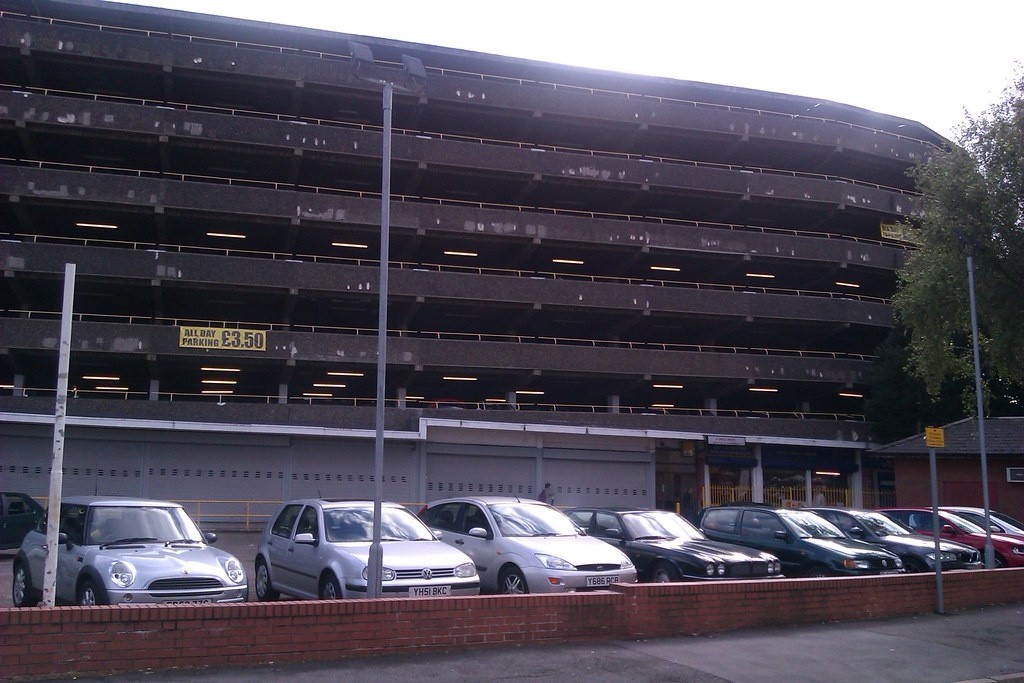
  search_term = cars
[254,497,481,601]
[557,506,787,586]
[415,494,639,596]
[11,496,250,605]
[860,508,1024,568]
[690,501,907,578]
[0,491,45,549]
[796,505,984,574]
[927,505,1024,536]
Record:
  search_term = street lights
[351,41,427,600]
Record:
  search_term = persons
[538,483,554,502]
